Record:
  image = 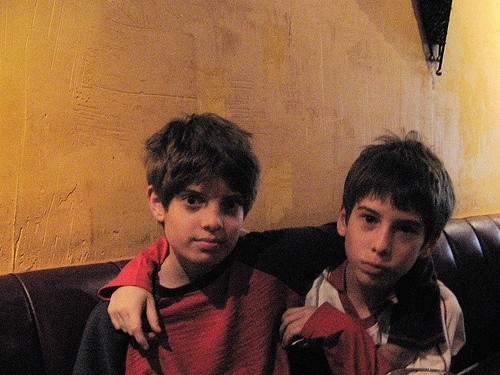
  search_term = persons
[98,137,466,375]
[72,112,302,375]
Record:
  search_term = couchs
[0,212,500,375]
